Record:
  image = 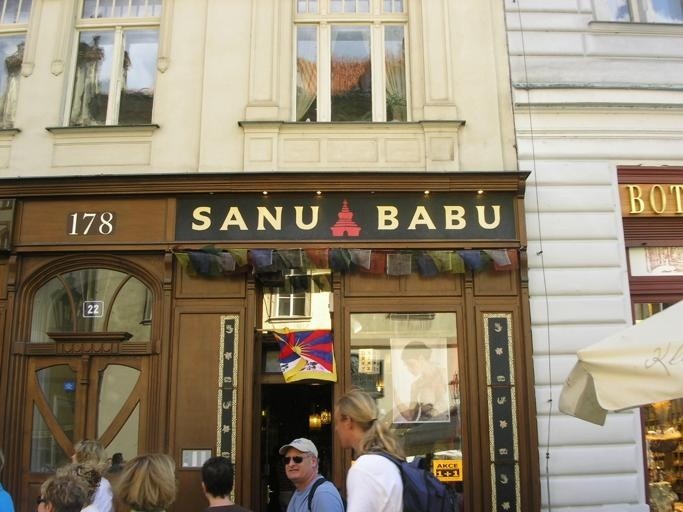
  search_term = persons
[37,439,175,512]
[392,340,447,421]
[1,451,16,512]
[280,438,345,512]
[201,457,253,512]
[334,389,409,512]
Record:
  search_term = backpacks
[356,450,458,512]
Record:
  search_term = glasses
[283,456,307,463]
[36,496,48,505]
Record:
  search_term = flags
[269,326,339,384]
[173,245,520,277]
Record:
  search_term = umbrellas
[556,297,682,426]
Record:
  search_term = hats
[278,438,319,459]
